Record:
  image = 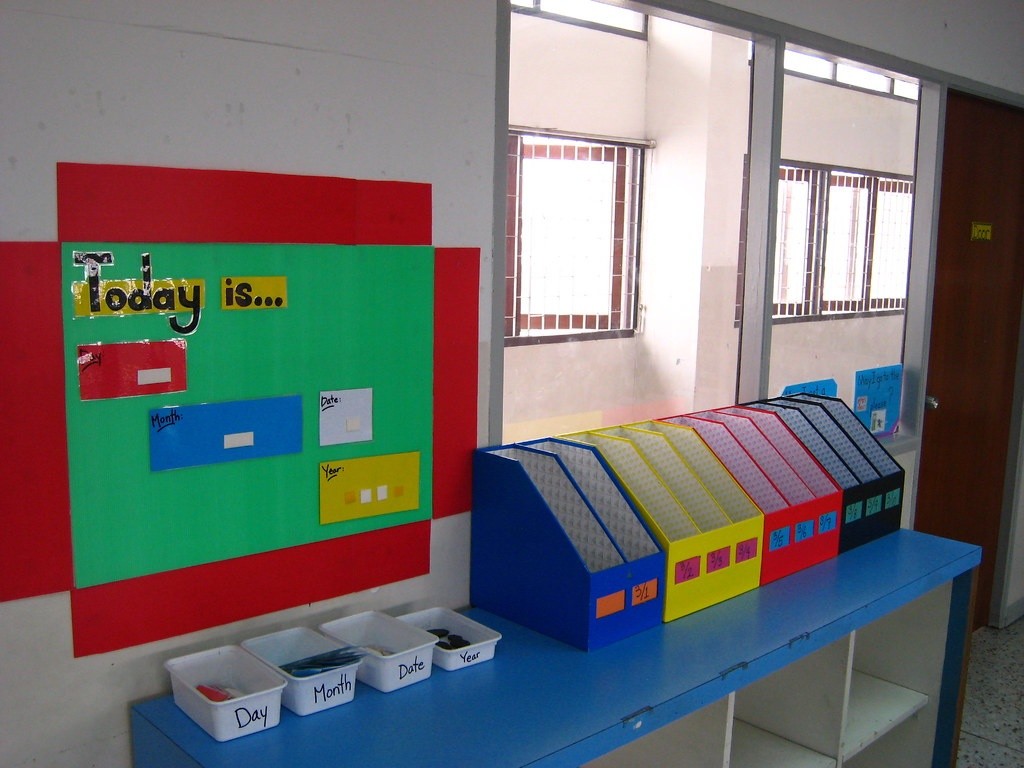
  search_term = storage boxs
[239,626,362,717]
[317,608,440,694]
[395,606,502,671]
[163,645,288,742]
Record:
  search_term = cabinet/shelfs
[128,528,985,768]
